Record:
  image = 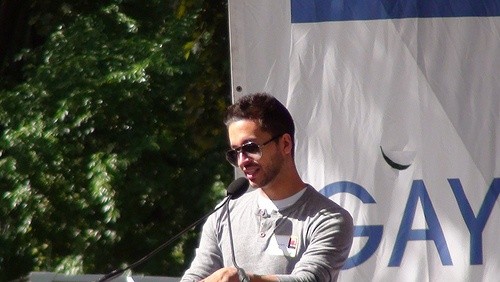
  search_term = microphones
[99,177,249,282]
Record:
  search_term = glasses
[226,137,276,168]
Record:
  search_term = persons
[176,93,355,282]
